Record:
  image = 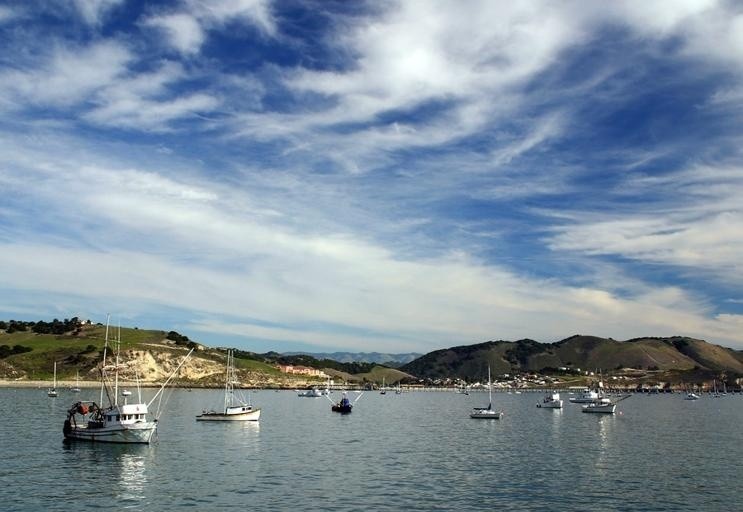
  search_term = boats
[298,386,353,413]
[684,392,701,400]
[536,388,617,413]
[469,364,501,418]
[380,376,402,394]
[455,389,469,394]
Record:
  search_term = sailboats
[195,349,261,421]
[48,313,194,444]
